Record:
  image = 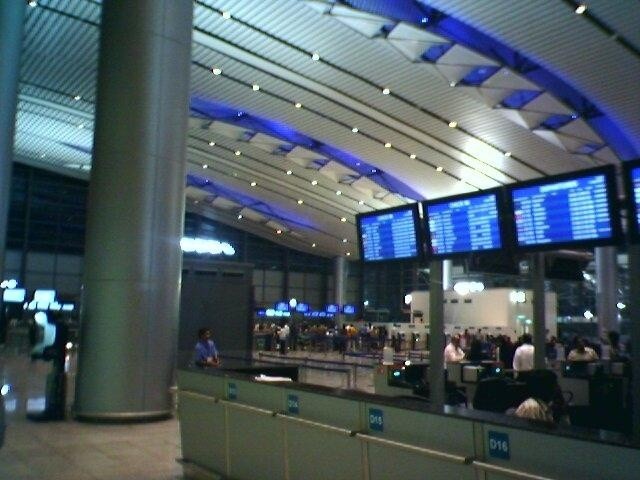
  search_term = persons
[511,367,571,427]
[254,322,388,356]
[443,328,630,381]
[197,327,219,366]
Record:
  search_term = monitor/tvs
[622,159,640,240]
[422,186,509,262]
[503,164,624,250]
[356,203,424,266]
